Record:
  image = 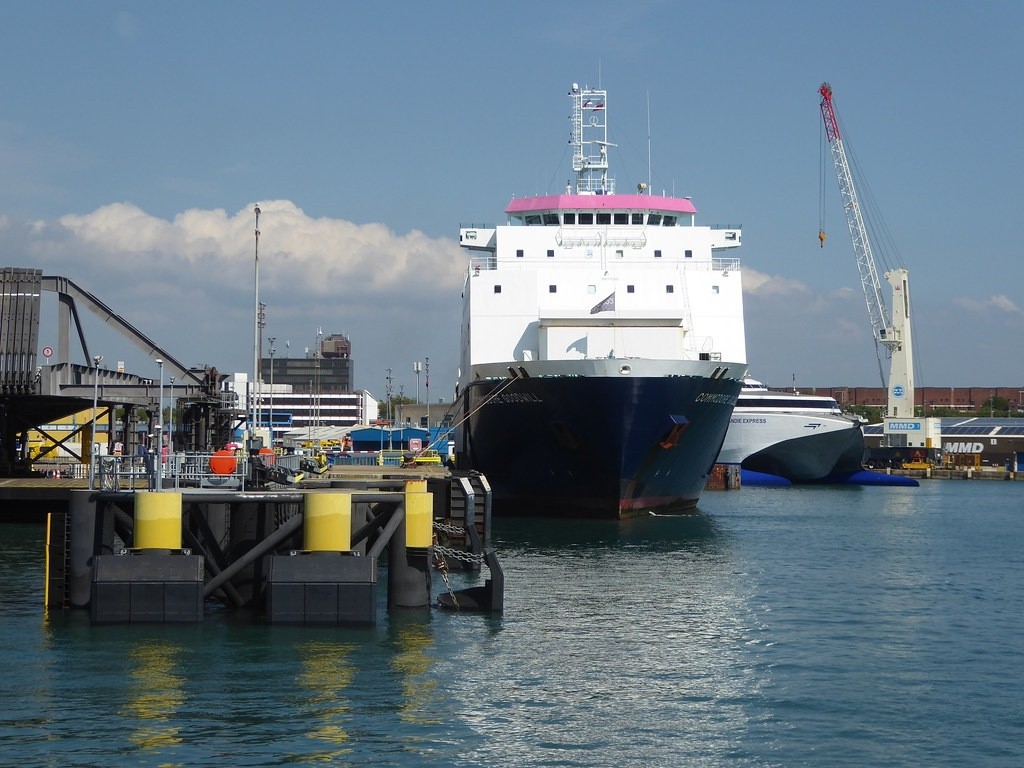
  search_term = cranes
[816,81,941,470]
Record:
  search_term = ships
[451,57,749,523]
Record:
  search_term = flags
[583,100,603,111]
[590,291,615,314]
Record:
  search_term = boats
[715,370,919,488]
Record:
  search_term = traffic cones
[52,470,61,480]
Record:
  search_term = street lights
[252,201,262,439]
[266,337,276,428]
[412,362,422,405]
[90,354,104,490]
[155,358,163,492]
[314,325,323,395]
[169,376,178,454]
